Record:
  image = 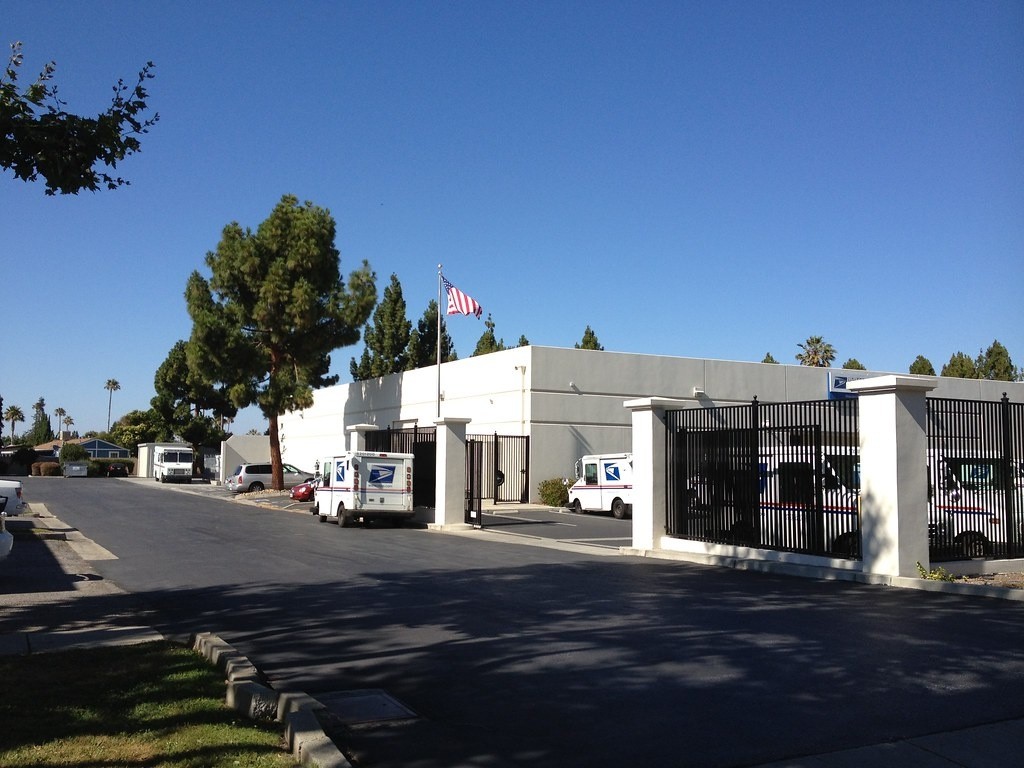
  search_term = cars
[106,463,129,478]
[289,481,316,502]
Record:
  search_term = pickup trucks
[0,478,29,517]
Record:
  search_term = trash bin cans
[63,462,88,477]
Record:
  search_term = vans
[709,443,957,564]
[153,445,194,484]
[926,445,1022,562]
[312,452,415,528]
[567,453,633,520]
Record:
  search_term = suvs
[230,462,317,494]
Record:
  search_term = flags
[441,276,482,320]
